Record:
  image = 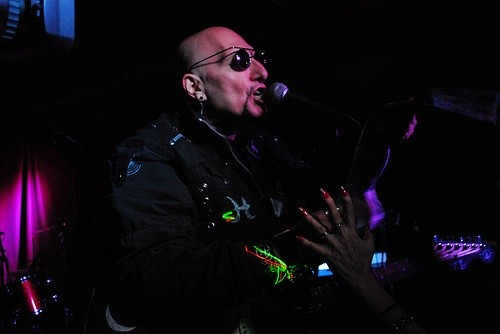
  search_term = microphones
[268,81,359,125]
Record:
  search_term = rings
[321,230,329,238]
[334,222,344,228]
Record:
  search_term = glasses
[187,47,268,72]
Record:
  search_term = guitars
[82,234,499,334]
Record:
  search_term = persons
[91,23,354,334]
[287,177,500,334]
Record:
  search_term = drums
[5,269,66,330]
